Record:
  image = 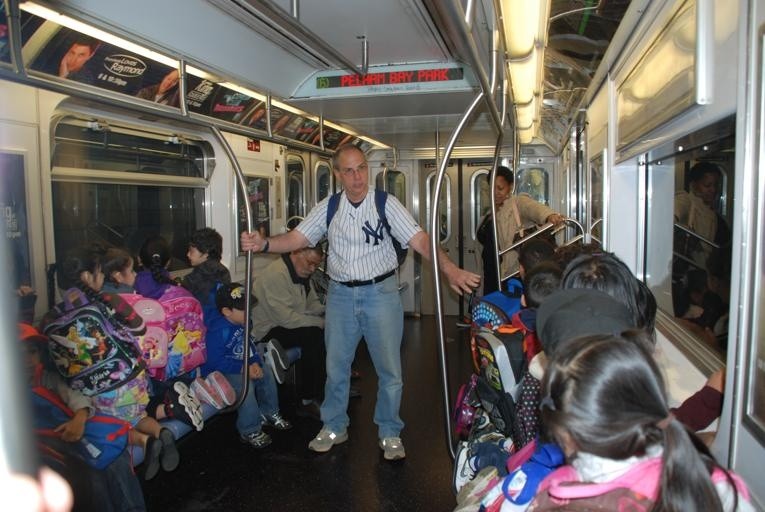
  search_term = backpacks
[469,322,538,436]
[327,189,408,265]
[526,455,748,512]
[45,279,207,397]
[469,278,525,330]
[29,383,134,472]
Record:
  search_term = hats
[215,282,258,309]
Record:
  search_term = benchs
[123,386,221,468]
[284,346,302,364]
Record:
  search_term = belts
[340,269,395,287]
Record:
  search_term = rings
[557,213,561,217]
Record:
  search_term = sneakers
[265,337,291,370]
[308,427,349,453]
[241,429,274,449]
[158,427,180,471]
[265,348,286,385]
[378,435,405,461]
[142,436,163,482]
[166,370,237,432]
[453,441,499,505]
[258,411,293,430]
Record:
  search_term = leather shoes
[294,398,322,421]
[349,386,363,399]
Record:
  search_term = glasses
[343,164,367,176]
[300,252,321,268]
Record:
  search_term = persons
[136,69,180,109]
[250,241,361,422]
[241,143,481,461]
[41,37,101,86]
[476,165,568,297]
[15,226,294,512]
[241,108,266,129]
[671,161,731,357]
[452,237,759,511]
[271,114,291,137]
